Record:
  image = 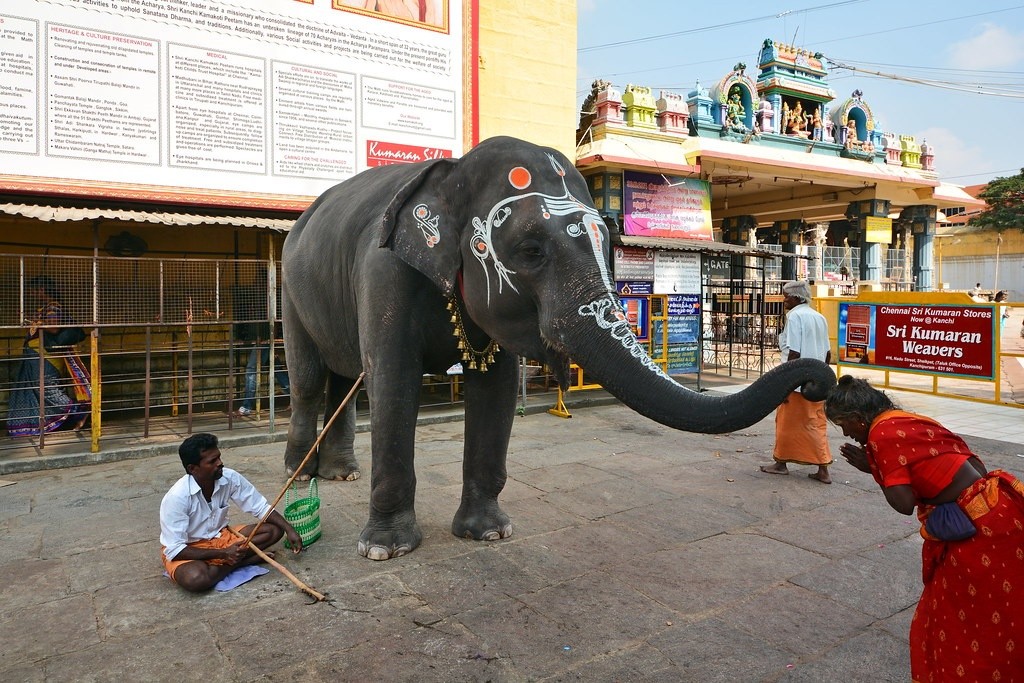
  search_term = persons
[822,373,1024,683]
[969,281,1024,347]
[760,280,834,485]
[159,433,304,592]
[579,38,937,171]
[225,274,297,418]
[8,275,92,437]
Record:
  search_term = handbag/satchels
[41,306,88,352]
[283,477,322,549]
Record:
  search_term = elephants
[279,136,837,561]
